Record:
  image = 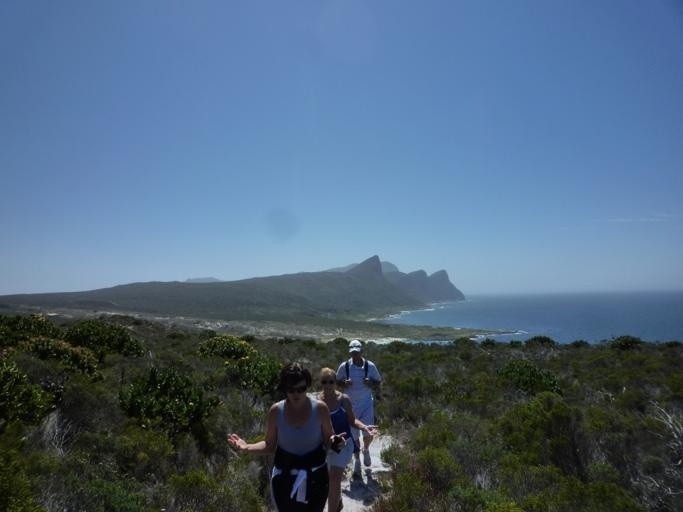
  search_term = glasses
[288,385,308,394]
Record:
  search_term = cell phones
[330,435,342,454]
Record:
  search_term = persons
[227,362,347,512]
[312,367,380,512]
[336,340,382,474]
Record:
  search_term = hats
[348,340,363,353]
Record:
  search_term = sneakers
[354,446,371,473]
